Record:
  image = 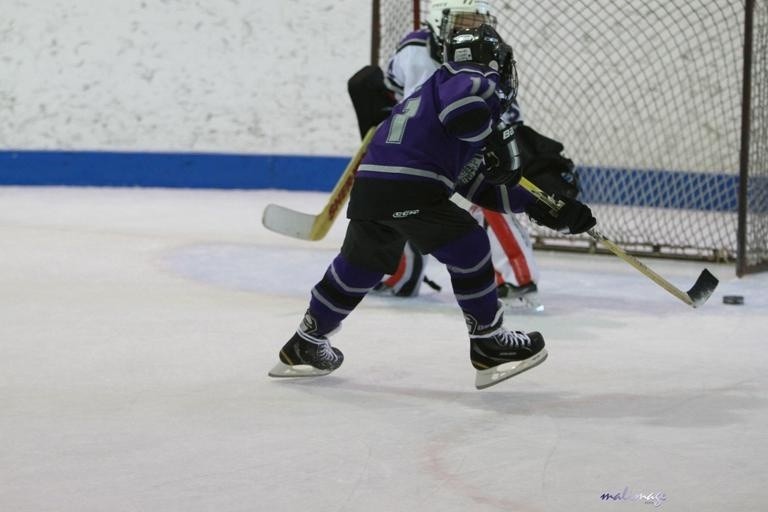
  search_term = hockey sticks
[482,151,719,311]
[262,120,384,241]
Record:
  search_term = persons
[280,23,597,371]
[347,0,582,302]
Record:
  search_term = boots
[279,308,343,371]
[462,296,546,371]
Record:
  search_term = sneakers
[497,281,541,300]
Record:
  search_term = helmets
[446,23,519,108]
[424,0,498,47]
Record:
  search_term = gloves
[482,117,524,188]
[524,190,597,235]
